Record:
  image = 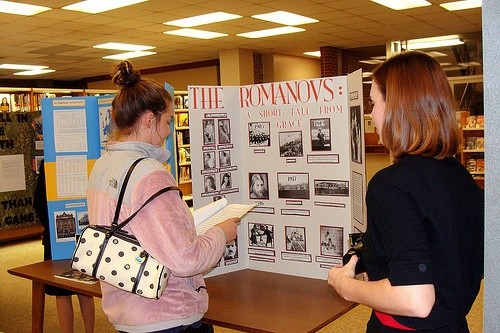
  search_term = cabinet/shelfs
[460,111,484,187]
[166,90,193,202]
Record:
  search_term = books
[191,198,256,236]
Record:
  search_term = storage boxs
[463,115,485,173]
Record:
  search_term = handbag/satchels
[69,157,183,300]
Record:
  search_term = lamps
[402,33,465,51]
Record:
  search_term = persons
[203,119,337,260]
[34,159,52,262]
[327,51,484,333]
[86,60,241,333]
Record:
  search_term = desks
[7,258,361,333]
